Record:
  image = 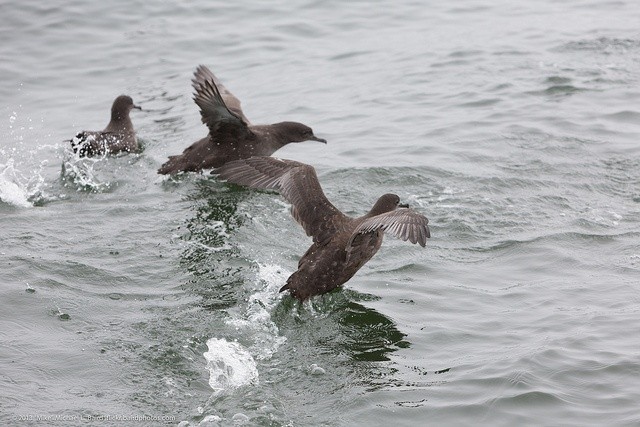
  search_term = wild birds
[158,63,327,173]
[69,93,143,158]
[210,156,429,300]
